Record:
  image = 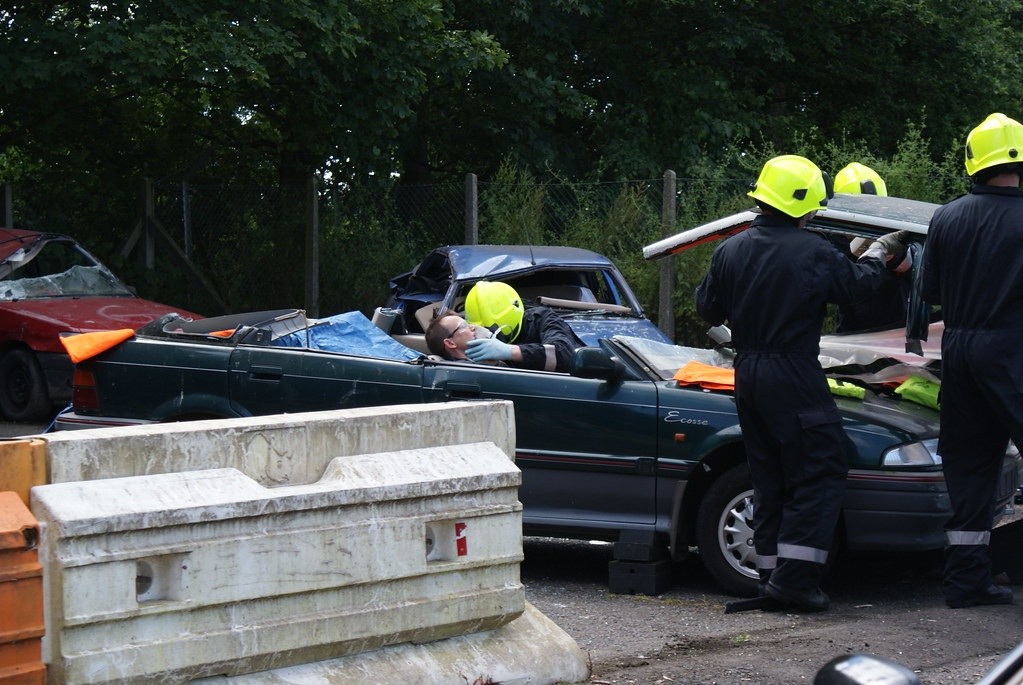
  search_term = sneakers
[944,583,1014,607]
[758,579,830,612]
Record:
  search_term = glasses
[443,320,467,348]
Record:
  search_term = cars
[642,196,948,394]
[1,227,208,424]
[54,240,1023,601]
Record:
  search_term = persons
[831,161,916,336]
[464,280,585,374]
[425,311,509,367]
[692,155,909,613]
[903,112,1023,610]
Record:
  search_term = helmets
[464,281,524,344]
[746,154,828,219]
[965,113,1023,176]
[833,162,888,197]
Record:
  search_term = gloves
[856,229,908,267]
[464,338,512,362]
